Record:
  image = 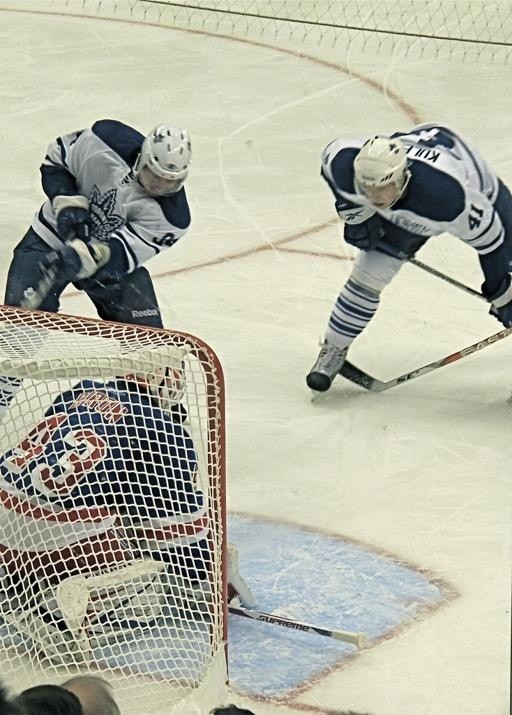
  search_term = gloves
[344,215,385,250]
[56,206,94,241]
[482,273,512,329]
[38,245,82,286]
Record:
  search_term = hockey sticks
[339,329,511,393]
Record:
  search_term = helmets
[122,344,189,415]
[132,122,194,199]
[351,135,412,213]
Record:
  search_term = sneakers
[26,596,70,656]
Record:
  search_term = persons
[0,360,259,668]
[3,118,191,329]
[0,675,121,715]
[306,122,511,392]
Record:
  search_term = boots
[304,338,348,394]
